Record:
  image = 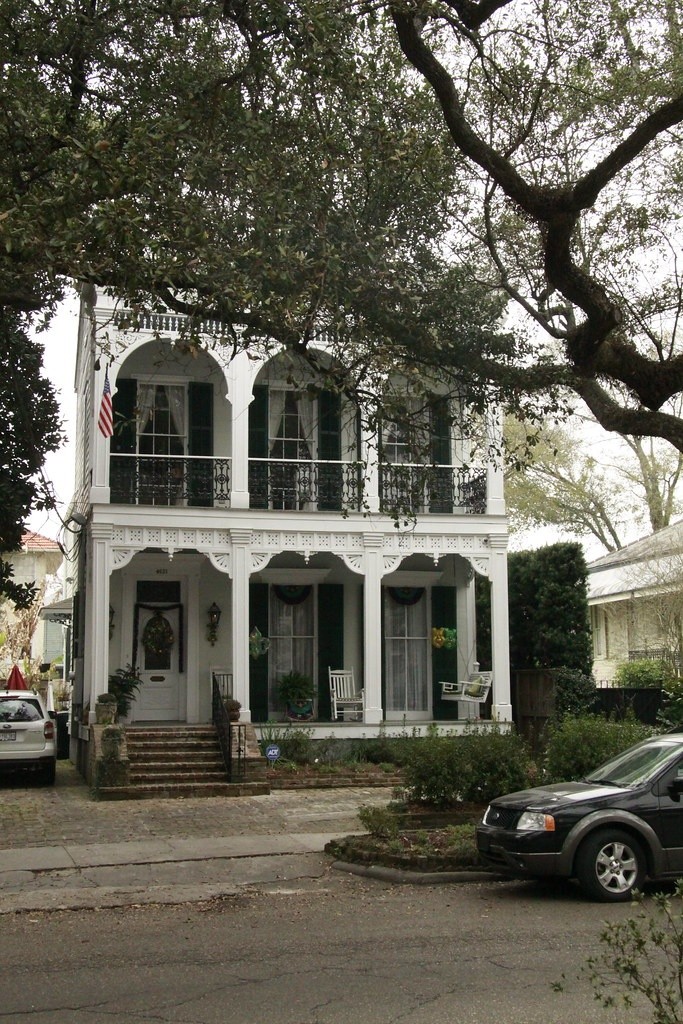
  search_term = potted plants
[97,728,131,786]
[94,694,118,724]
[225,699,242,722]
[273,670,318,720]
[52,683,71,711]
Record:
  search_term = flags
[97,363,114,439]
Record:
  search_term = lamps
[206,601,224,646]
[109,604,115,640]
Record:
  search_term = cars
[477,732,683,904]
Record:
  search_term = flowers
[142,620,173,654]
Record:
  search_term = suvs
[0,687,58,785]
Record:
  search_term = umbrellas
[5,665,29,691]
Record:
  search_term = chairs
[328,664,365,722]
